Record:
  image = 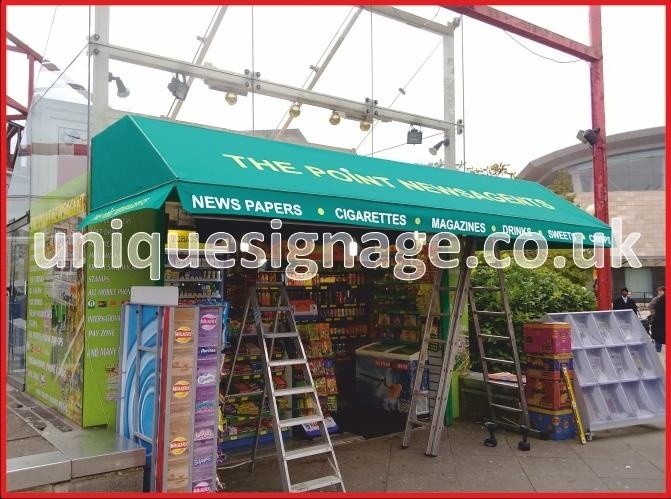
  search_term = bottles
[257,258,368,354]
[164,257,220,305]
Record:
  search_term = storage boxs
[525,377,575,411]
[527,406,576,441]
[522,321,572,359]
[526,354,573,381]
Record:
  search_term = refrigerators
[355,341,429,421]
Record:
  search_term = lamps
[167,72,189,101]
[429,138,450,155]
[108,72,130,97]
[576,127,601,146]
[407,124,422,144]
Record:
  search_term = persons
[655,287,665,351]
[647,289,660,338]
[627,291,641,319]
[613,288,636,315]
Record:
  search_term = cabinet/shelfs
[537,308,665,440]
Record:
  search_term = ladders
[402,236,532,457]
[216,281,347,492]
